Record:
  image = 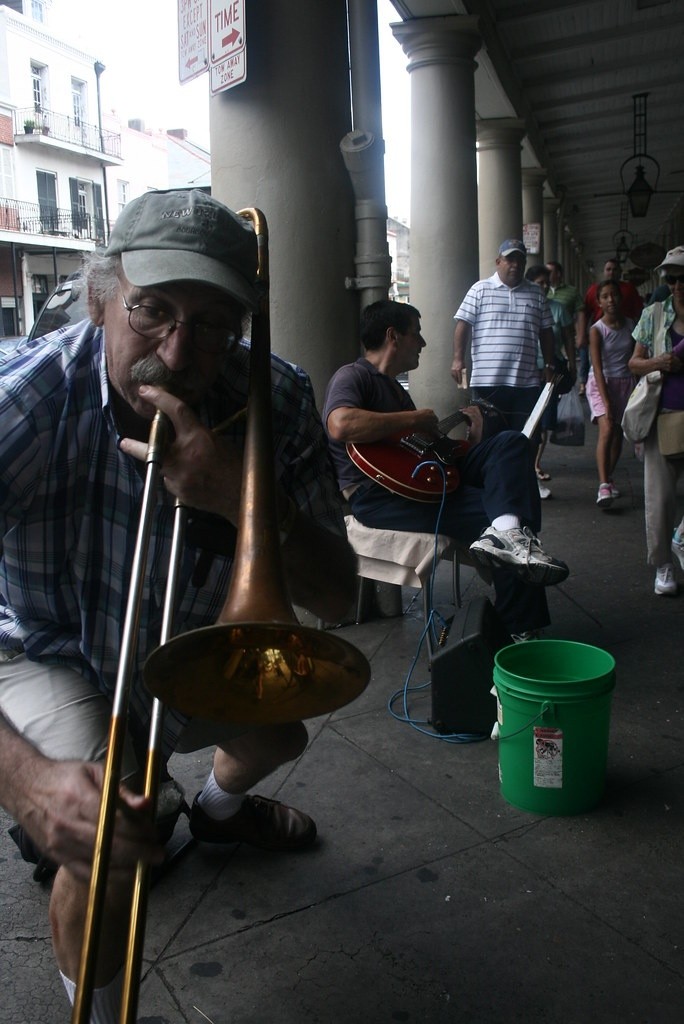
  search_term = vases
[42,130,48,135]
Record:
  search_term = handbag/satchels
[621,370,663,443]
[549,384,585,447]
[656,411,684,459]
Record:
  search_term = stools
[343,514,460,672]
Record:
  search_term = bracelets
[546,364,555,370]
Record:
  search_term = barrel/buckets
[490,639,618,816]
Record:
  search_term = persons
[584,279,637,507]
[0,190,360,1024]
[526,265,576,480]
[323,300,570,641]
[628,246,684,595]
[578,347,589,397]
[584,259,644,324]
[451,239,555,499]
[648,285,671,307]
[546,261,588,351]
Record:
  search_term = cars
[24,269,91,343]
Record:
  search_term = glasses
[661,274,684,285]
[114,272,241,356]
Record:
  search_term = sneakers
[670,528,684,571]
[189,791,318,852]
[468,527,569,587]
[510,633,537,643]
[654,563,677,595]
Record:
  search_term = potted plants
[24,120,35,133]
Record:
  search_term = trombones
[71,206,374,1024]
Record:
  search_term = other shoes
[595,487,614,507]
[578,388,585,396]
[609,480,619,497]
[535,468,550,481]
[537,481,552,500]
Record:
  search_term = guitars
[345,393,503,505]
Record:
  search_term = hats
[498,239,528,257]
[104,189,259,315]
[653,246,684,272]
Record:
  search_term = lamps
[620,93,659,217]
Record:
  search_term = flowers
[43,127,49,130]
